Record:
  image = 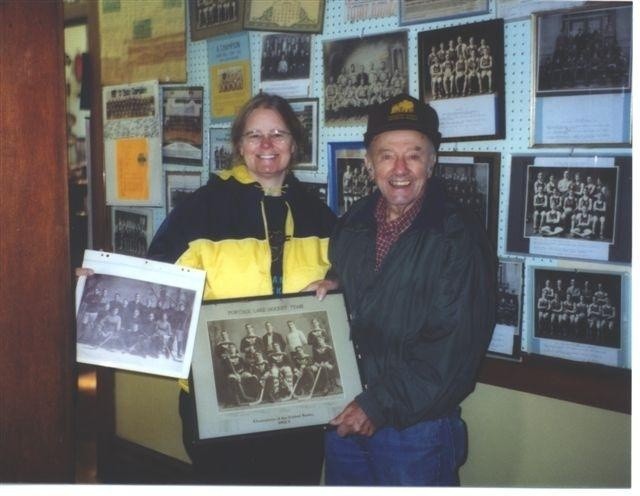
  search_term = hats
[362,93,441,138]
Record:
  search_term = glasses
[244,129,292,143]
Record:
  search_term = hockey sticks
[229,356,258,401]
[299,368,323,400]
[280,378,300,402]
[250,384,265,406]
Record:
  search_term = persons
[428,37,494,98]
[326,63,406,118]
[550,28,627,87]
[215,319,337,405]
[77,282,188,362]
[343,164,375,211]
[530,170,609,240]
[537,276,616,336]
[499,286,517,326]
[300,92,499,486]
[436,172,483,216]
[214,145,233,169]
[75,93,338,484]
[262,34,309,76]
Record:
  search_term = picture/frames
[187,0,250,41]
[165,170,202,216]
[326,141,378,215]
[242,0,326,35]
[191,289,368,444]
[286,97,319,170]
[400,0,631,371]
